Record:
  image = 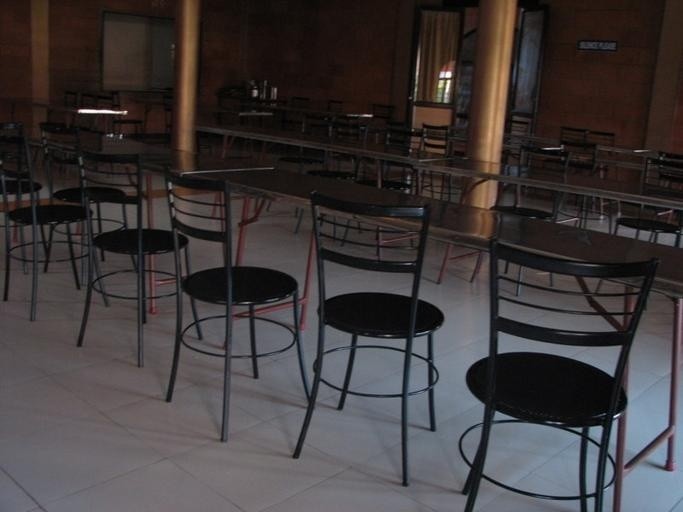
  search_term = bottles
[249,79,279,101]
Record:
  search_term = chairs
[161,170,315,443]
[292,190,445,487]
[457,235,662,508]
[0,84,204,367]
[206,84,683,328]
